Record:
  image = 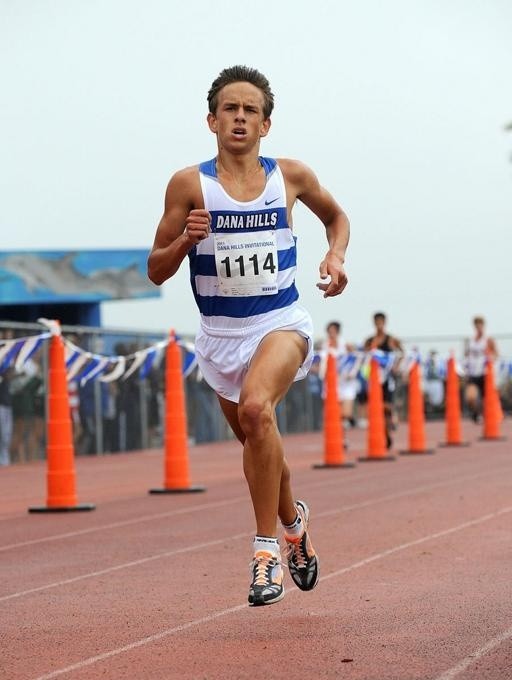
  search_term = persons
[463,316,499,423]
[309,322,361,452]
[362,313,405,450]
[146,62,351,607]
